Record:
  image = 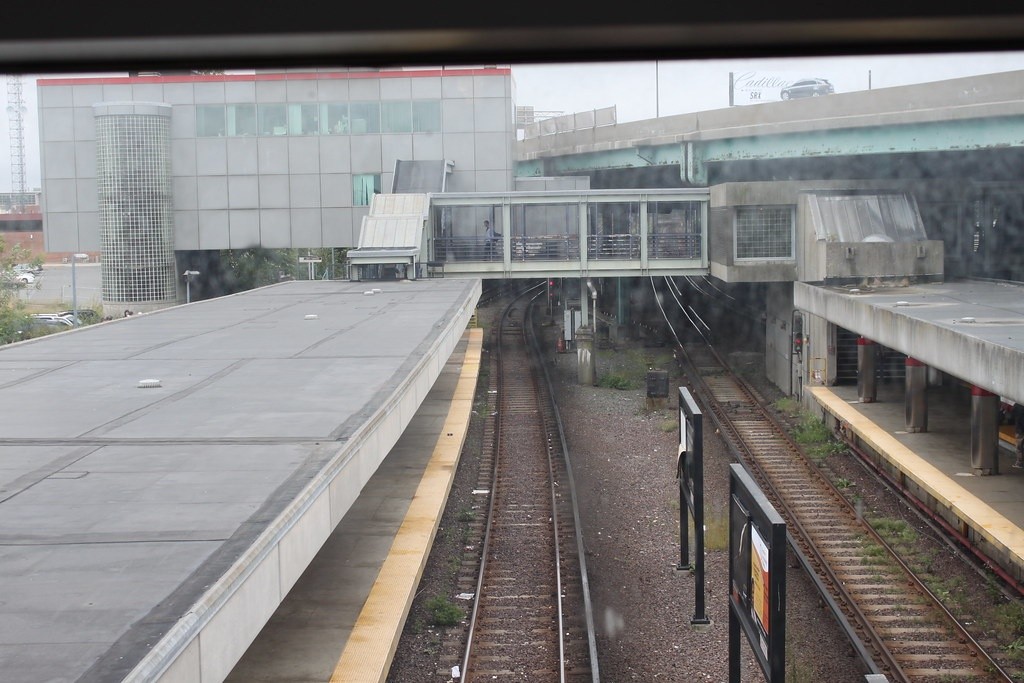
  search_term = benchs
[999,426,1017,452]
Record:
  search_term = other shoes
[1012,461,1023,468]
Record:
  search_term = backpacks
[493,232,501,243]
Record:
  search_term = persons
[483,220,499,261]
